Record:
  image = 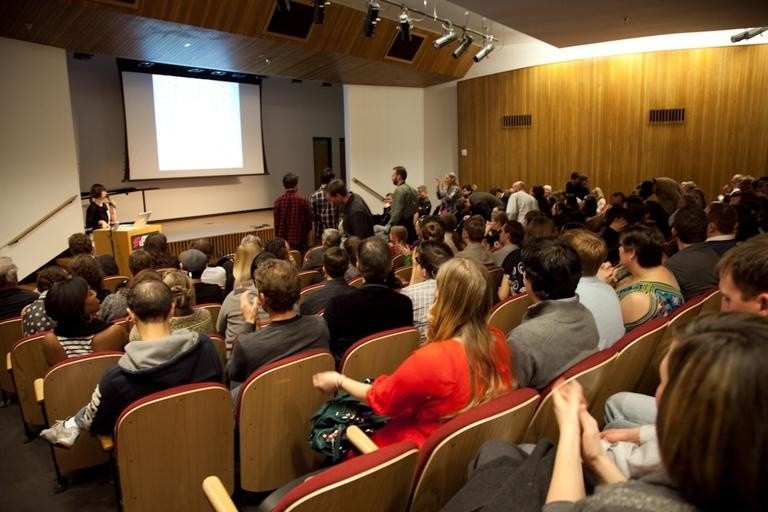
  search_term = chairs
[0,246,720,512]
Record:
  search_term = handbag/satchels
[308,376,397,458]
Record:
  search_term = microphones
[105,195,116,208]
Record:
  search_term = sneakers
[40,416,82,450]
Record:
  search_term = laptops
[135,212,151,226]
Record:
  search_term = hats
[179,249,208,271]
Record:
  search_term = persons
[519,235,767,475]
[84,184,116,257]
[543,331,767,512]
[273,166,766,387]
[312,257,513,459]
[1,233,329,448]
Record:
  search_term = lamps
[313,0,498,63]
[731,25,768,43]
